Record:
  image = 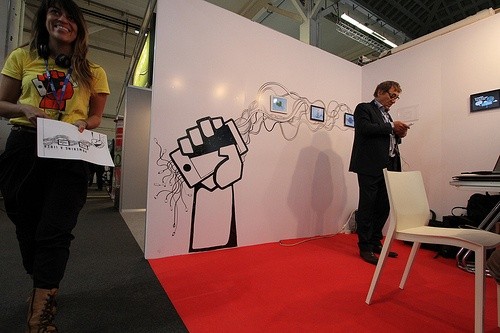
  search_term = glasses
[386,91,400,101]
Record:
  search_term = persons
[87,161,105,192]
[348,80,411,265]
[0,1,111,333]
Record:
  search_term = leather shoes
[360,249,378,265]
[374,242,398,257]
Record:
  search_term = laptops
[461,156,500,174]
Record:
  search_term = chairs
[366,168,500,333]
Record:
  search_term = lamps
[341,11,399,48]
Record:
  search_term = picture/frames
[344,113,355,128]
[470,89,500,112]
[270,95,288,114]
[310,105,324,122]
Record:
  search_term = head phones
[39,43,72,68]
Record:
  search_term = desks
[449,181,500,277]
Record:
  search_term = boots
[26,288,60,333]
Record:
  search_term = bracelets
[79,119,88,129]
